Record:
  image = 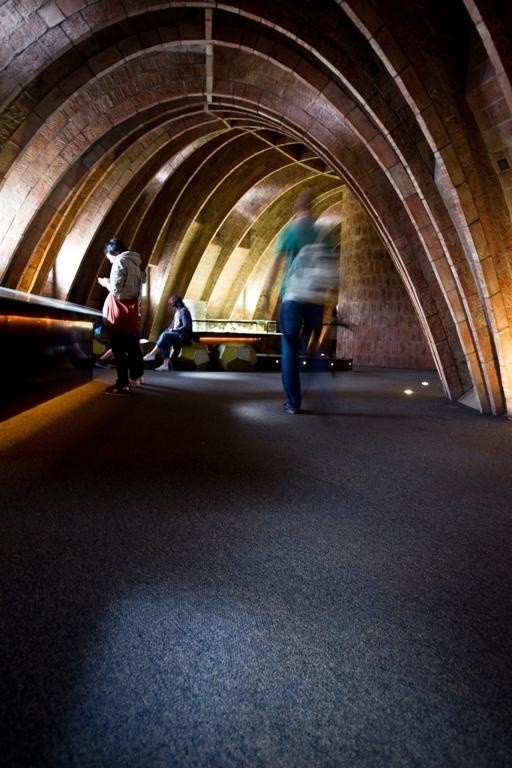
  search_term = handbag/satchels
[102,292,139,336]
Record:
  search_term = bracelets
[259,294,267,297]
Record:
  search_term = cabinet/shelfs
[191,319,281,356]
[0,285,102,427]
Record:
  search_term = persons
[143,294,192,371]
[257,190,338,414]
[94,238,144,396]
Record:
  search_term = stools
[168,344,211,371]
[211,342,259,372]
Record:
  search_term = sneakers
[95,359,117,369]
[143,353,155,361]
[106,377,144,395]
[283,399,300,415]
[155,364,168,372]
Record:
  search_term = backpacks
[285,240,340,305]
